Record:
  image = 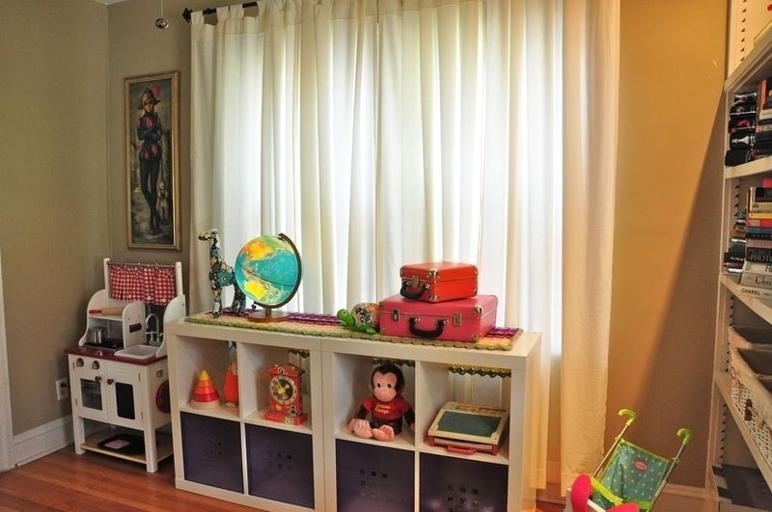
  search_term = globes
[234,233,302,322]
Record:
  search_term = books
[721,177,772,297]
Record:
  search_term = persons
[136,88,164,235]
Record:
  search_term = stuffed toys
[198,227,249,322]
[336,302,379,335]
[350,359,415,443]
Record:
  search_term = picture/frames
[120,72,183,253]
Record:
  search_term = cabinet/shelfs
[64,349,171,475]
[707,25,771,510]
[321,339,527,512]
[168,325,325,512]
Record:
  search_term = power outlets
[54,376,70,400]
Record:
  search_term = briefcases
[378,261,498,343]
